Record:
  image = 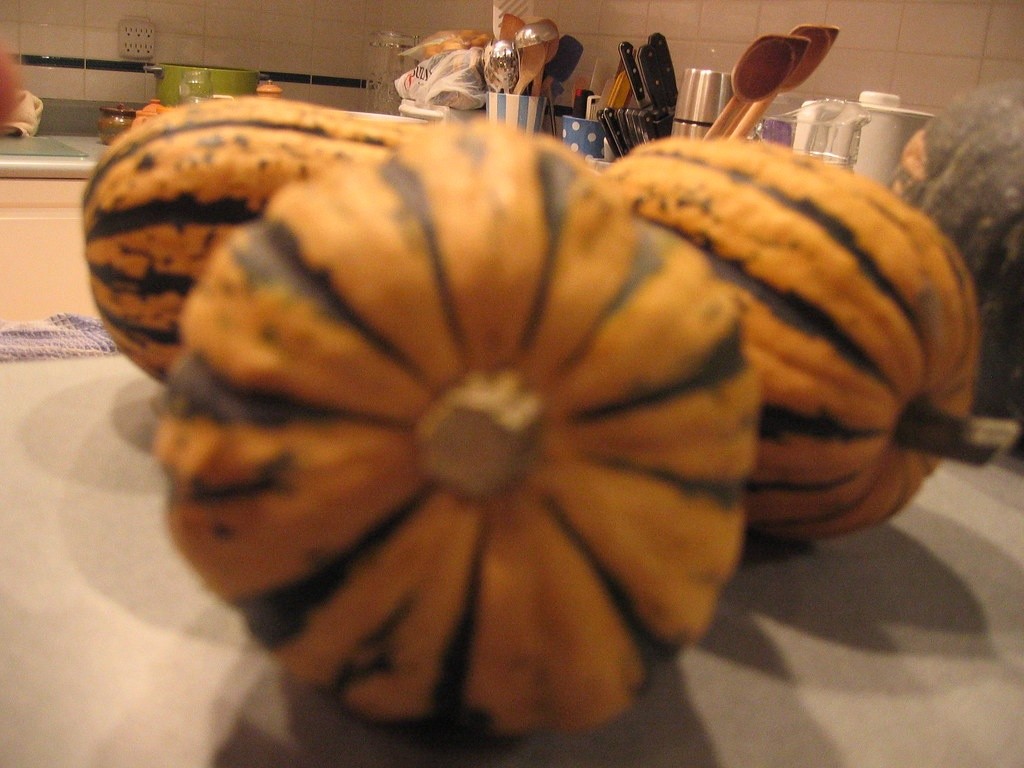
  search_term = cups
[366,32,419,115]
[562,116,605,167]
[486,92,547,136]
[180,70,213,104]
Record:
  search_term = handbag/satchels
[395,48,487,111]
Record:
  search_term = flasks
[671,69,734,141]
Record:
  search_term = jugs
[748,99,871,172]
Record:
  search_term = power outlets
[117,19,155,60]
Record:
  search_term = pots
[143,63,270,108]
[794,91,935,191]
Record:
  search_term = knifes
[597,33,679,157]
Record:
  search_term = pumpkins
[82,80,1024,742]
